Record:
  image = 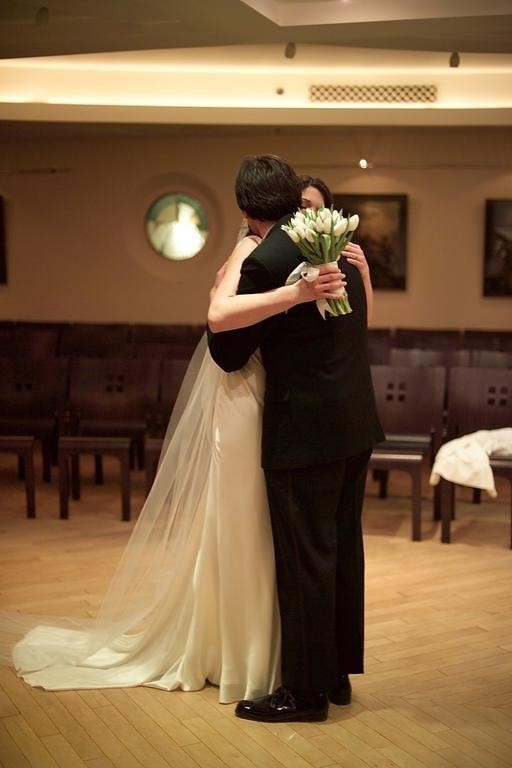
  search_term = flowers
[284,207,362,319]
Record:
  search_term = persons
[173,174,374,698]
[204,153,384,726]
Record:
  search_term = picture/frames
[329,193,410,289]
[481,198,512,299]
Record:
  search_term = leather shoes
[325,673,351,704]
[236,685,327,722]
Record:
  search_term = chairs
[364,325,511,543]
[2,318,206,524]
